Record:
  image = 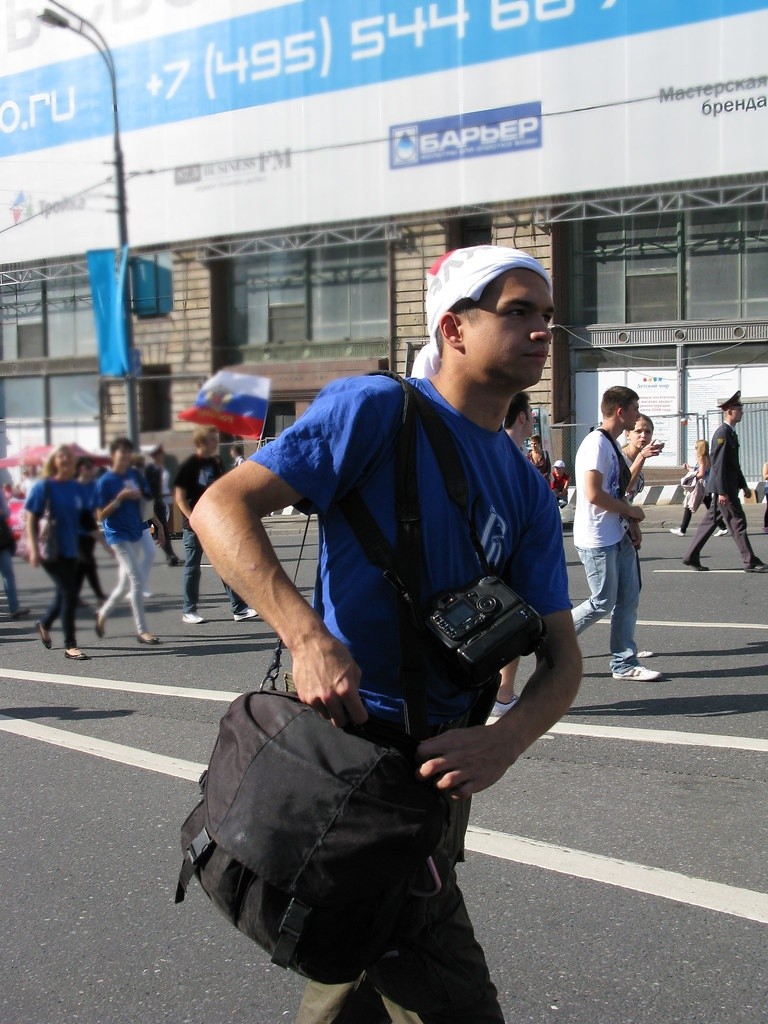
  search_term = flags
[178,369,272,440]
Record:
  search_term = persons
[762,462,768,533]
[189,245,582,1024]
[489,386,665,718]
[682,390,768,573]
[670,440,729,537]
[0,424,258,660]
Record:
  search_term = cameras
[421,573,547,676]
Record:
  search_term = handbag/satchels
[14,477,57,563]
[589,426,632,498]
[174,692,450,985]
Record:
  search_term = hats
[552,460,565,468]
[411,246,553,379]
[716,391,744,409]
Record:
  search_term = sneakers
[490,695,518,717]
[612,665,662,681]
[713,528,729,537]
[10,607,30,618]
[182,612,205,624]
[636,648,653,658]
[670,528,686,537]
[167,553,185,567]
[234,608,258,622]
[143,590,152,598]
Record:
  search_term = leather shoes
[745,563,768,573]
[682,559,709,571]
[64,650,88,660]
[34,619,52,649]
[92,609,105,639]
[136,634,160,645]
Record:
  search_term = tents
[0,443,112,468]
